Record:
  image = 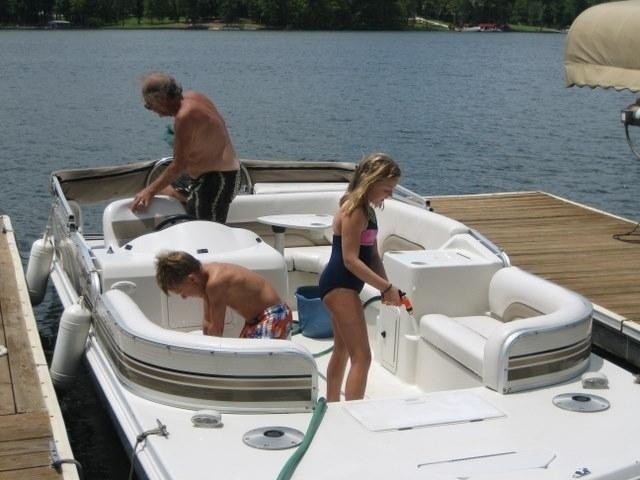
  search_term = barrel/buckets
[293,283,335,340]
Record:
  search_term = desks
[258,208,346,250]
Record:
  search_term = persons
[317,150,401,401]
[153,250,293,342]
[129,74,242,225]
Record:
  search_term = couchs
[94,190,468,250]
[420,267,590,398]
[96,294,323,414]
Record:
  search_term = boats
[455,24,502,32]
[24,155,639,480]
